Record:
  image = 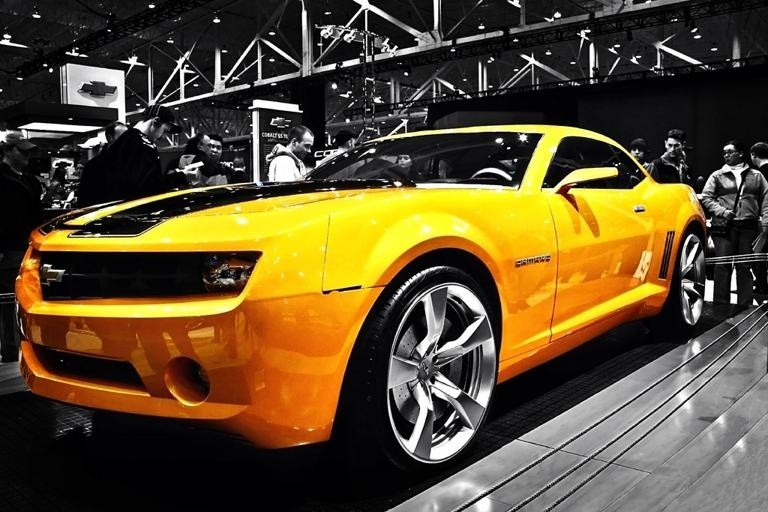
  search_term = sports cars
[12,121,713,488]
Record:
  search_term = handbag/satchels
[708,213,733,237]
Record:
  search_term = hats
[0,133,40,152]
[141,104,184,135]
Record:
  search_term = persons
[323,128,359,158]
[76,122,128,208]
[393,155,414,179]
[183,133,246,188]
[99,105,198,202]
[24,157,43,195]
[76,159,87,176]
[92,144,102,155]
[630,129,767,320]
[1,131,53,363]
[266,125,314,182]
